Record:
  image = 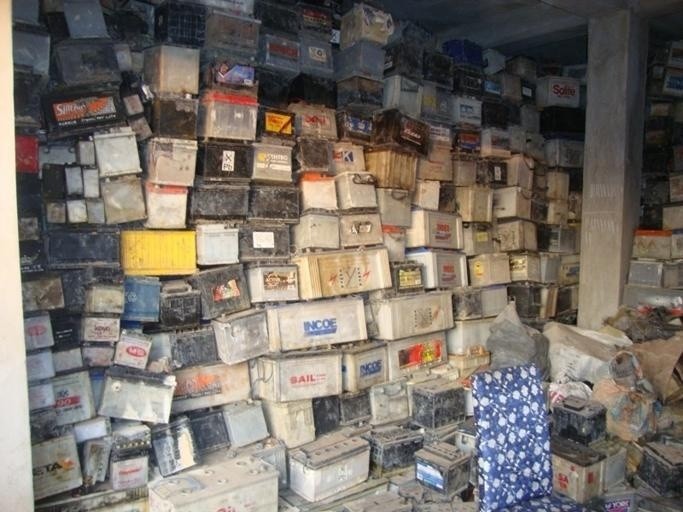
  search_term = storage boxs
[0,0,683,510]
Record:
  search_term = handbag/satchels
[593,350,658,442]
